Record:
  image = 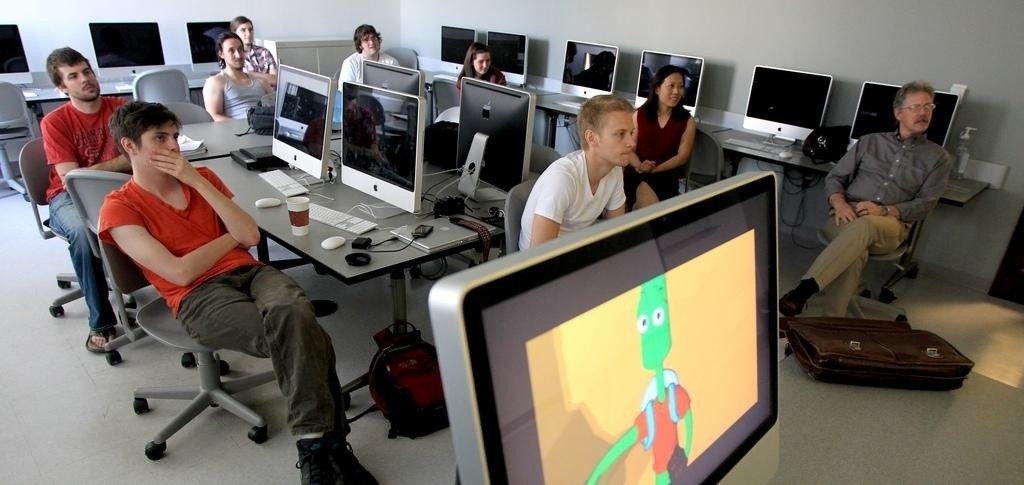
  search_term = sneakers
[294,437,333,485]
[323,425,379,485]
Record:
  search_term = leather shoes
[779,290,805,317]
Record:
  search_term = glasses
[901,103,935,111]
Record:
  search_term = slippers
[86,328,116,353]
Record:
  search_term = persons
[455,41,507,102]
[301,117,324,156]
[778,79,953,316]
[201,31,274,122]
[38,45,132,356]
[93,98,383,483]
[342,85,386,165]
[624,64,697,210]
[217,15,278,87]
[517,93,638,252]
[337,23,401,92]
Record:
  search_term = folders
[230,146,289,170]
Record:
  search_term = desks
[715,369,1023,484]
[911,172,992,279]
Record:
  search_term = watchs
[879,205,891,216]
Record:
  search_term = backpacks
[368,321,449,438]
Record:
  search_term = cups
[286,196,310,237]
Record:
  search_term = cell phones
[411,223,434,238]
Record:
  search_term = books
[177,136,208,157]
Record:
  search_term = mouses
[779,151,793,159]
[320,236,346,251]
[255,198,281,208]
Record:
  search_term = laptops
[390,214,496,254]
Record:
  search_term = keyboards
[115,82,132,92]
[433,73,459,83]
[23,91,38,98]
[553,98,582,110]
[724,137,786,155]
[258,168,310,197]
[309,201,378,236]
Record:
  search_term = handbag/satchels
[779,317,974,390]
[802,126,851,165]
[248,94,274,135]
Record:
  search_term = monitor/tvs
[846,81,961,167]
[743,64,835,148]
[88,22,167,79]
[634,48,707,121]
[486,30,530,85]
[360,58,427,97]
[338,81,427,215]
[270,63,337,186]
[426,167,783,485]
[561,39,620,105]
[440,25,477,73]
[454,76,537,204]
[0,24,36,84]
[185,21,231,73]
